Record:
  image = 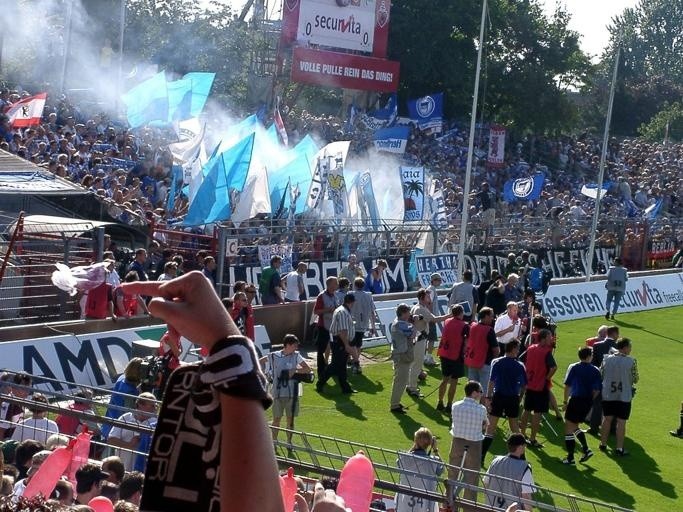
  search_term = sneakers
[390,356,629,464]
[670,431,683,439]
[316,358,361,393]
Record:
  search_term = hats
[431,273,441,282]
[76,466,110,481]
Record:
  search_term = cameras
[410,314,424,323]
[292,373,315,383]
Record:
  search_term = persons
[0,68,682,512]
